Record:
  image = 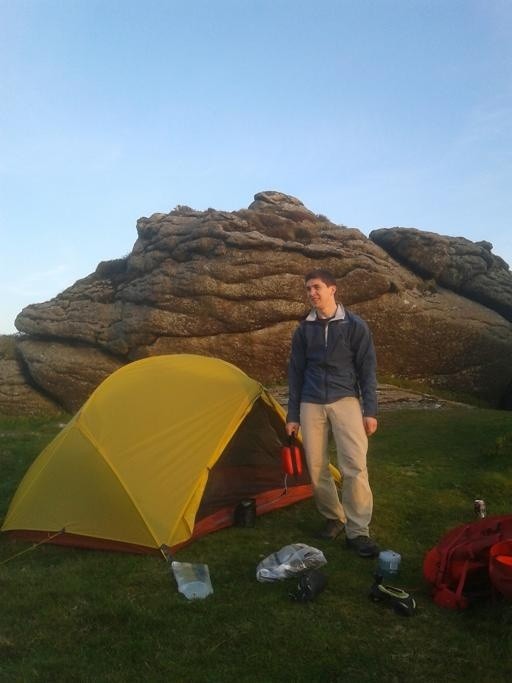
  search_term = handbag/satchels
[423,514,512,609]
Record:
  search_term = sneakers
[311,519,345,542]
[344,535,382,557]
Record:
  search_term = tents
[0,353,343,562]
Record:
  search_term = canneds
[474,500,487,520]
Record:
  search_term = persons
[284,269,380,557]
